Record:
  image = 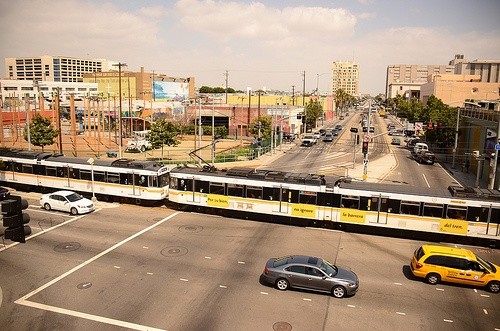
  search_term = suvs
[416,151,435,165]
[410,244,500,294]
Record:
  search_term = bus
[125,130,156,153]
[0,147,170,207]
[169,141,500,246]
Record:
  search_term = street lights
[112,63,129,159]
[255,89,265,155]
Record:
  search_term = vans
[413,143,428,155]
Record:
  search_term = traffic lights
[1,195,31,243]
[363,141,368,154]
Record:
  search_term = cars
[301,108,402,147]
[263,255,360,299]
[407,139,419,149]
[39,190,95,216]
